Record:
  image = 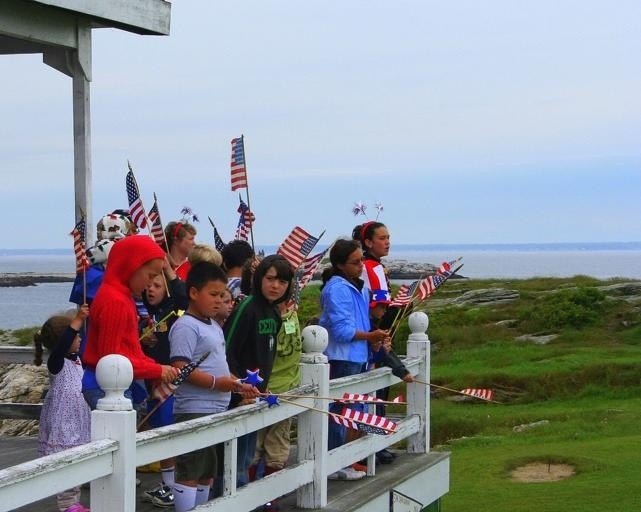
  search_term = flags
[65,133,461,436]
[460,389,494,401]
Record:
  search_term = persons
[34,209,417,512]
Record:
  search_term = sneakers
[144,481,176,508]
[375,449,394,467]
[64,503,90,511]
[328,463,367,482]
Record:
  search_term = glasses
[345,256,365,265]
